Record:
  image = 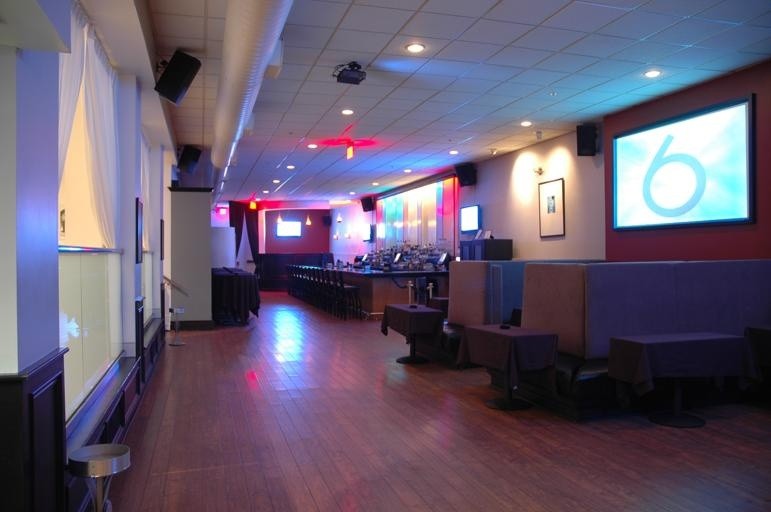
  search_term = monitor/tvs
[362,224,372,241]
[275,219,303,238]
[459,204,481,233]
[612,92,756,231]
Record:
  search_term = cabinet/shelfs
[459,239,513,262]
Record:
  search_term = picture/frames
[538,179,566,238]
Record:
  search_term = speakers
[177,144,202,174]
[361,197,374,211]
[577,125,596,155]
[323,215,331,225]
[154,50,201,105]
[456,163,477,186]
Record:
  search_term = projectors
[337,69,366,84]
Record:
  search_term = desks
[606,330,744,429]
[212,266,260,326]
[384,302,558,416]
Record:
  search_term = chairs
[287,264,362,321]
